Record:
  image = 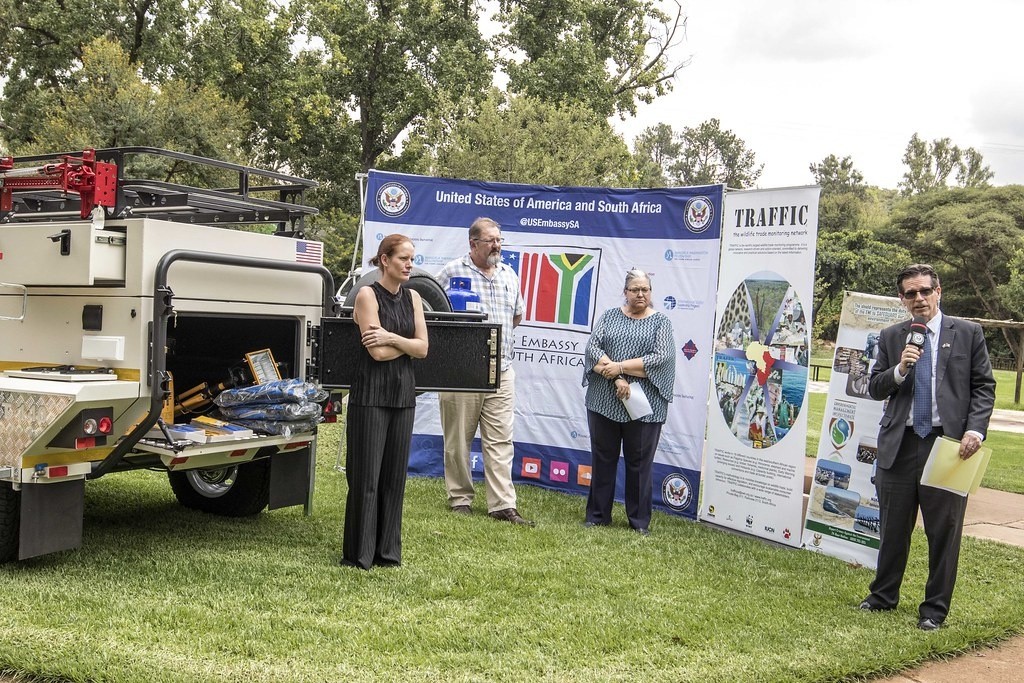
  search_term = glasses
[903,286,935,299]
[626,286,650,296]
[474,238,504,244]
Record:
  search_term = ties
[913,334,932,438]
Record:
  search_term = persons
[774,298,808,366]
[857,447,877,462]
[434,218,534,527]
[717,387,794,437]
[583,269,676,536]
[857,264,997,629]
[342,234,429,570]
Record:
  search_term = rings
[971,448,974,451]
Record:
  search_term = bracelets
[612,375,622,383]
[620,362,623,374]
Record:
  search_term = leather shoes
[859,601,873,611]
[451,505,472,517]
[489,508,535,528]
[634,528,649,538]
[584,521,595,528]
[918,618,938,630]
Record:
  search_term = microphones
[906,317,927,368]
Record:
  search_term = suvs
[1,143,500,566]
